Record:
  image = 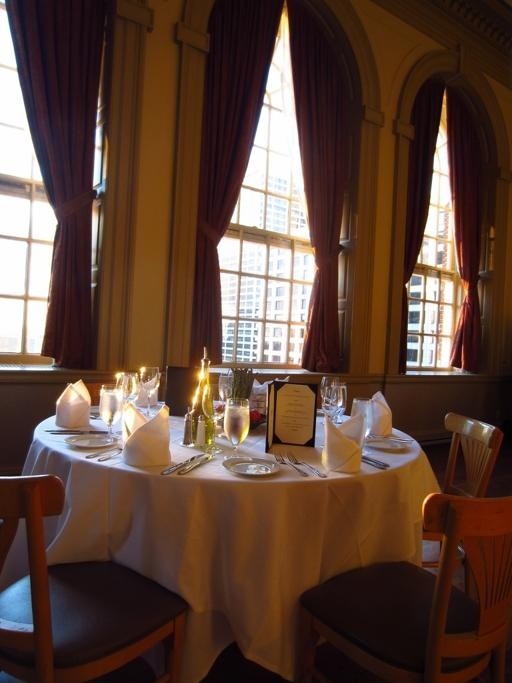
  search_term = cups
[115,370,141,403]
[218,372,235,401]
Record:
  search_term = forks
[272,449,328,478]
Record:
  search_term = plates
[364,438,410,451]
[223,458,282,477]
[64,434,118,449]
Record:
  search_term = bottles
[181,413,207,449]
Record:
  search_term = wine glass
[318,375,349,449]
[139,365,162,418]
[222,396,250,460]
[98,383,125,441]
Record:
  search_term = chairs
[1,472,191,683]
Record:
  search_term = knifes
[84,445,122,462]
[159,452,214,476]
